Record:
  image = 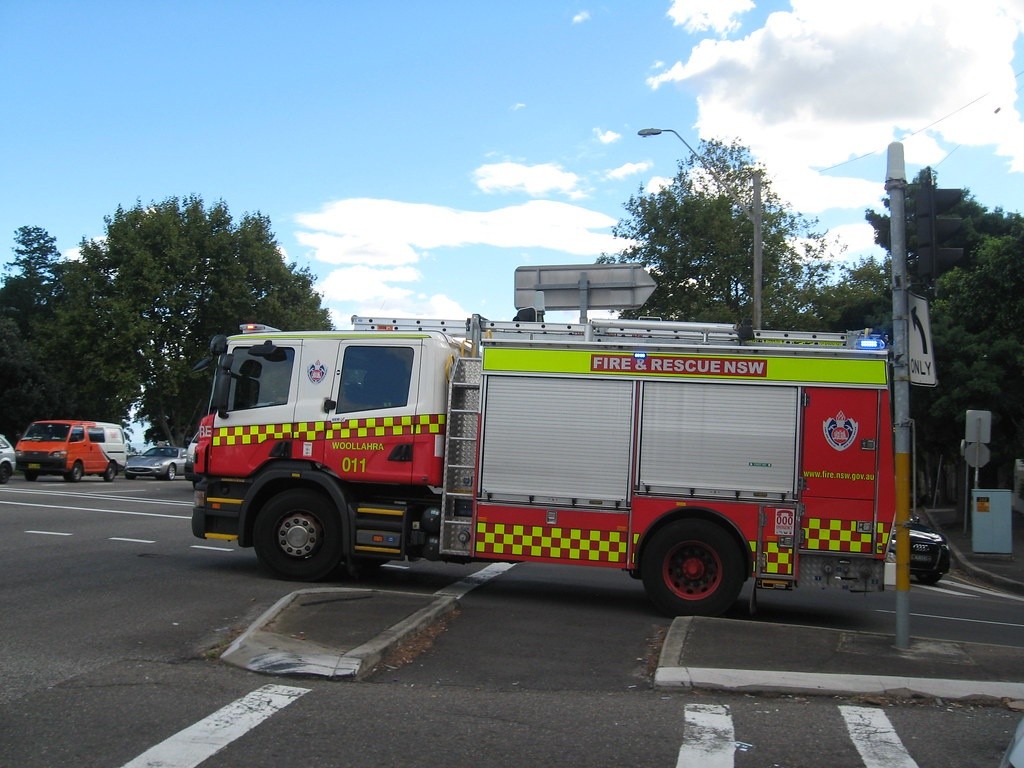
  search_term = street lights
[638,128,762,329]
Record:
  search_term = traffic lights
[913,165,964,279]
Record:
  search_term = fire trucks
[193,314,902,617]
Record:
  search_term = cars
[0,434,17,485]
[183,431,199,480]
[888,517,951,585]
[125,447,187,481]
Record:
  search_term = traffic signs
[906,291,936,387]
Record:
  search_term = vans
[15,420,127,483]
